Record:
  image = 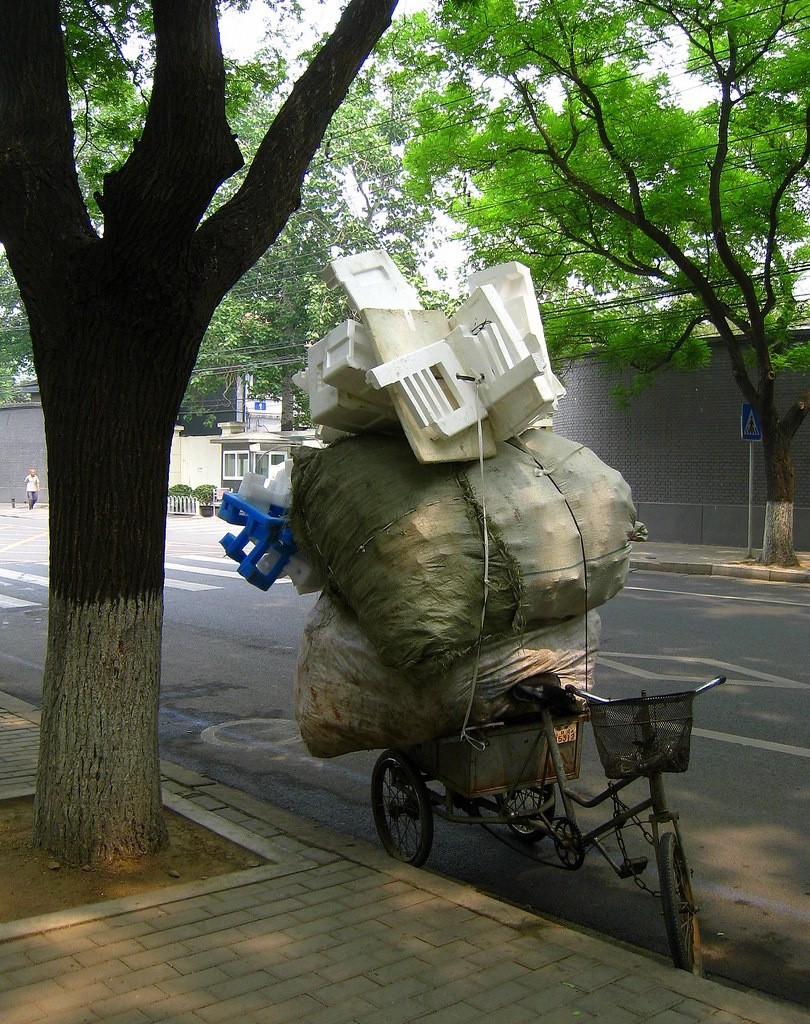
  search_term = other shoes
[29,505,33,510]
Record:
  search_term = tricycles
[369,668,728,978]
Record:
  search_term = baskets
[586,688,697,778]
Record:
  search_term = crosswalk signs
[740,403,763,443]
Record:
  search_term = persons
[23,469,40,510]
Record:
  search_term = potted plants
[168,484,193,515]
[191,485,217,517]
